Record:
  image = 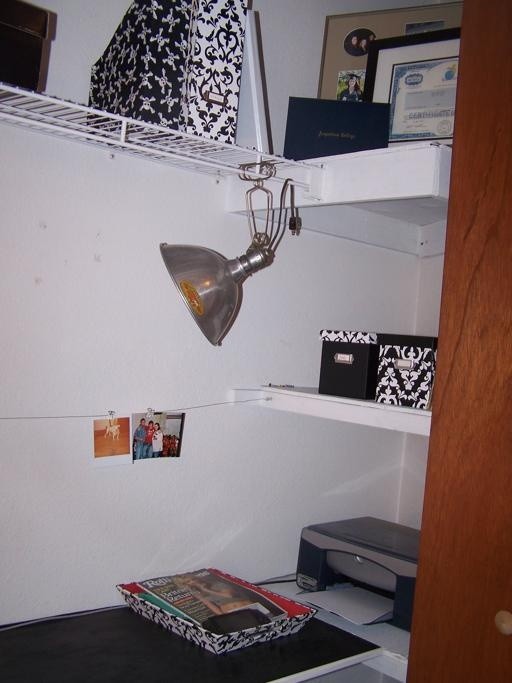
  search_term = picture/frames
[362,26,460,145]
[316,1,463,100]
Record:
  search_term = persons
[133,418,180,460]
[173,573,256,614]
[338,75,361,100]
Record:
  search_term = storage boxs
[374,334,439,411]
[318,329,378,410]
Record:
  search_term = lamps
[158,160,276,346]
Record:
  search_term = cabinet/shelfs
[1,0,512,682]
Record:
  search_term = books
[136,567,288,626]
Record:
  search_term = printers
[296,517,420,631]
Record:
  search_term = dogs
[105,424,120,441]
